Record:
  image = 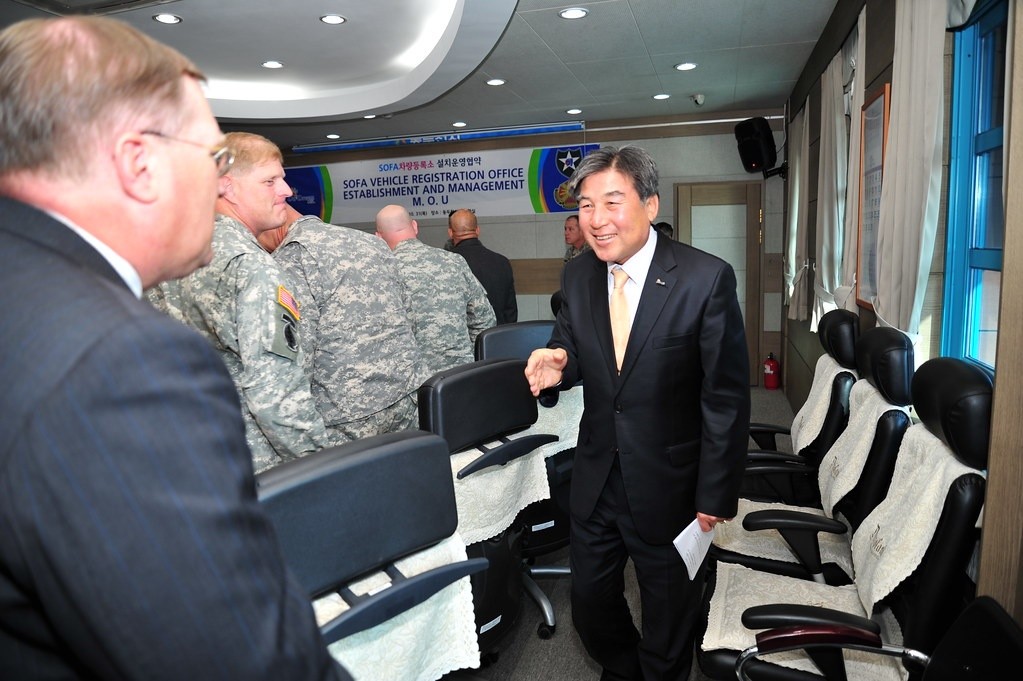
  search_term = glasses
[113,129,238,179]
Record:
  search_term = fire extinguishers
[763,351,779,390]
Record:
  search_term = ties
[609,268,630,376]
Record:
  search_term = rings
[720,520,726,525]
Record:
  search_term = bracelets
[555,380,562,388]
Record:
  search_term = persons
[448,209,518,326]
[257,201,432,446]
[375,205,497,375]
[524,146,751,681]
[443,210,458,250]
[143,132,331,474]
[562,215,593,264]
[656,222,673,240]
[0,15,354,681]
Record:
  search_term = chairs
[255,429,490,681]
[419,359,556,635]
[732,309,862,505]
[695,354,1000,679]
[706,323,923,585]
[474,319,584,556]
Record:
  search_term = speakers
[734,116,776,174]
